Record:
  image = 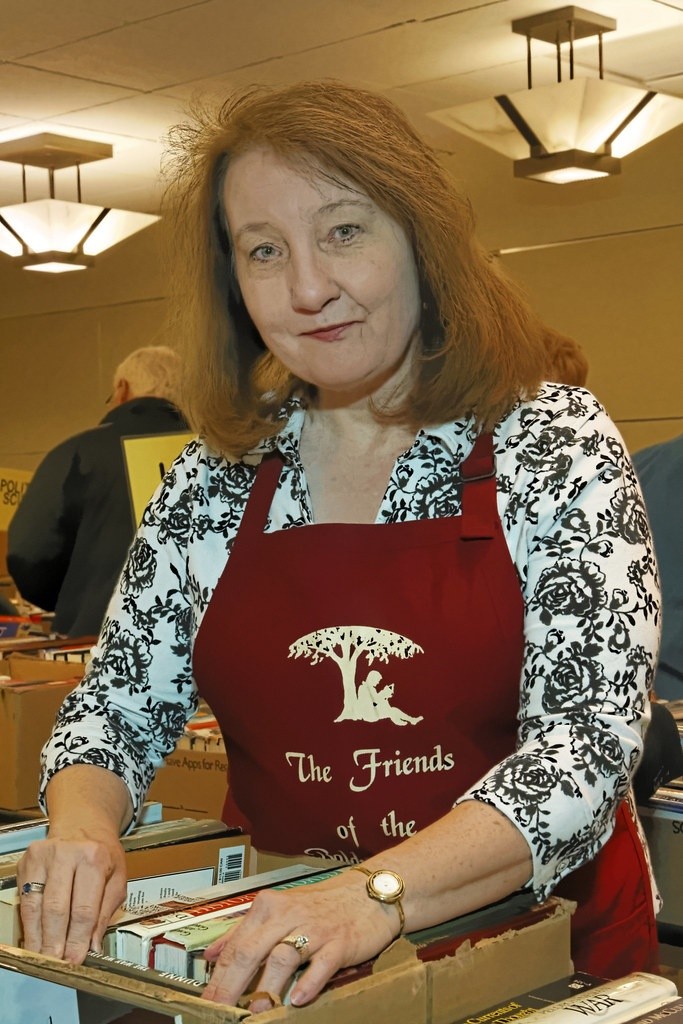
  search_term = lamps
[424,5,683,186]
[0,131,163,274]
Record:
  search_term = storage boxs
[0,651,87,812]
[145,737,228,822]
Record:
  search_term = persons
[6,346,192,638]
[630,434,683,701]
[17,82,664,1013]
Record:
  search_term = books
[0,646,229,751]
[0,800,683,1024]
[636,698,683,822]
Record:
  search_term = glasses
[104,388,116,407]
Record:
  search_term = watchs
[350,865,405,935]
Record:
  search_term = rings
[22,882,45,895]
[282,936,310,963]
